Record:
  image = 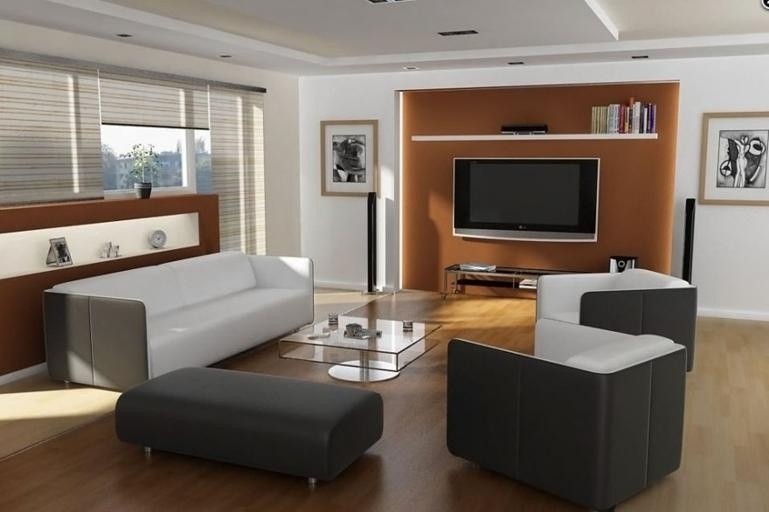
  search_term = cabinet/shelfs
[0,191,221,378]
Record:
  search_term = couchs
[42,251,315,394]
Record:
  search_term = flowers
[125,142,160,183]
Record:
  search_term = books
[459,262,497,273]
[591,96,657,133]
[519,279,538,289]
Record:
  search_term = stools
[115,366,385,486]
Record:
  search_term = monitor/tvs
[452,156,599,243]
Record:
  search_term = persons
[731,135,750,188]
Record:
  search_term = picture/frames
[319,119,379,197]
[44,238,73,269]
[698,111,769,207]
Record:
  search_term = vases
[133,182,152,198]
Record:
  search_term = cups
[328,312,338,326]
[402,319,413,331]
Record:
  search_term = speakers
[608,256,638,273]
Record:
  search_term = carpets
[0,286,394,470]
